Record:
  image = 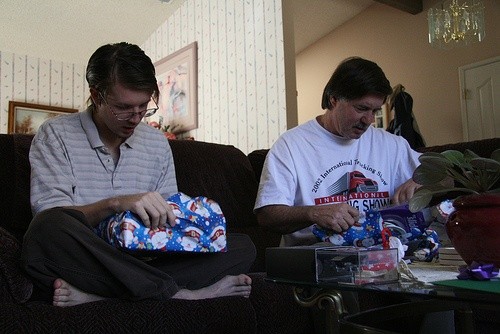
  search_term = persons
[253,56,424,247]
[22,42,257,307]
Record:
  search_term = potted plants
[408,147,500,270]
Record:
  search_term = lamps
[427,0,486,48]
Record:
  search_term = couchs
[0,133,438,334]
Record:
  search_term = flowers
[145,115,195,141]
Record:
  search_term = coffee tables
[264,275,500,334]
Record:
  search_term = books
[438,247,467,265]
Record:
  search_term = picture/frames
[143,42,198,134]
[9,100,79,135]
[370,101,389,130]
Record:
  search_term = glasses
[98,91,159,121]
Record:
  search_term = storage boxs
[265,243,399,287]
[369,202,435,232]
